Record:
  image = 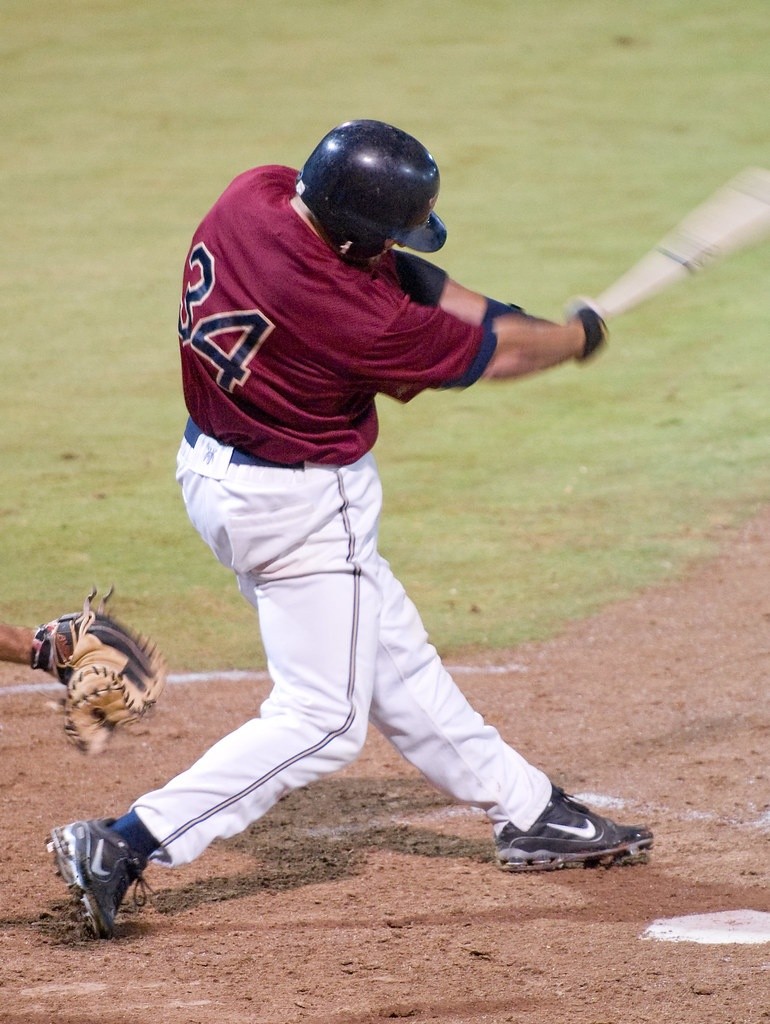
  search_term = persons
[44,117,653,938]
[0,584,166,756]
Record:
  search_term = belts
[182,418,306,469]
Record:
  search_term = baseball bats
[589,165,770,322]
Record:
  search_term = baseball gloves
[30,610,170,758]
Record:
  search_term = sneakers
[493,781,655,873]
[45,817,155,940]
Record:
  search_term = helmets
[296,119,448,253]
